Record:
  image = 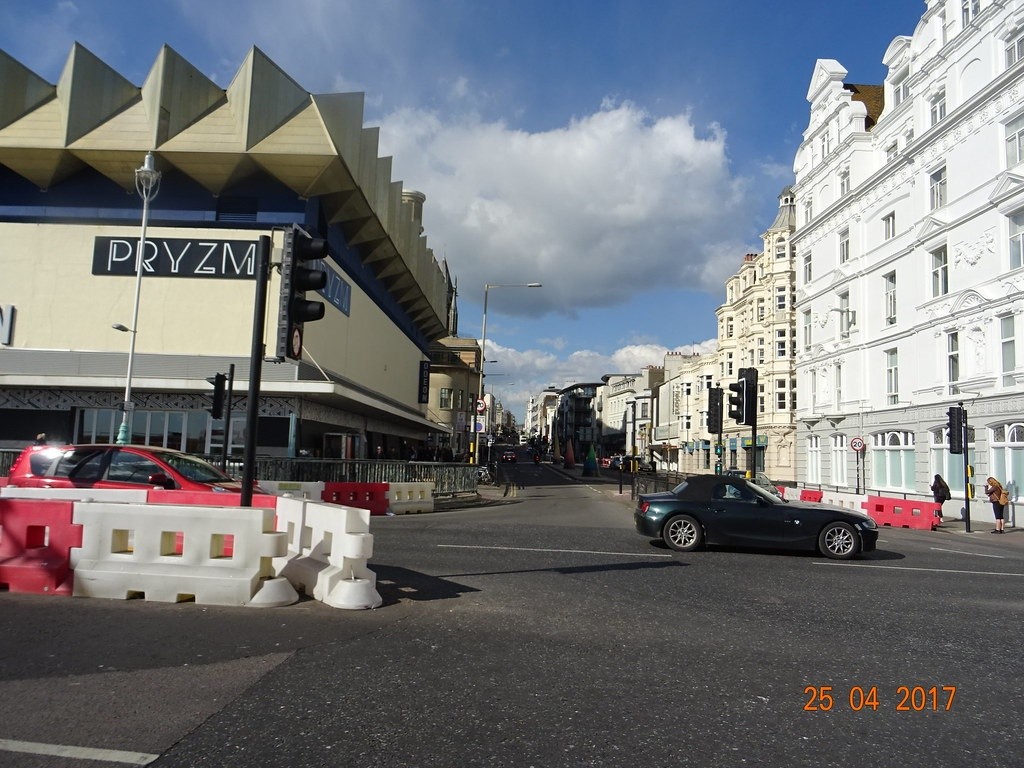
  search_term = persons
[984,477,1004,534]
[37,433,46,445]
[716,485,727,498]
[931,474,946,523]
[374,445,453,479]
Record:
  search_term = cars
[725,469,783,499]
[501,448,516,463]
[610,456,623,470]
[623,455,653,474]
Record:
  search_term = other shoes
[1000,530,1004,533]
[991,530,1001,534]
[940,517,944,523]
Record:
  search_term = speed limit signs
[851,438,864,451]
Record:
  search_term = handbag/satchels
[945,492,951,500]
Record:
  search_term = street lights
[626,400,636,474]
[486,382,515,462]
[115,149,161,448]
[467,282,542,462]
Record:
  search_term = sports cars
[634,473,879,561]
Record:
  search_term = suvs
[6,440,271,495]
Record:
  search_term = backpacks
[993,486,1009,506]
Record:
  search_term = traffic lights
[728,378,746,424]
[716,445,722,456]
[204,372,226,419]
[715,463,722,476]
[946,406,962,454]
[275,223,332,364]
